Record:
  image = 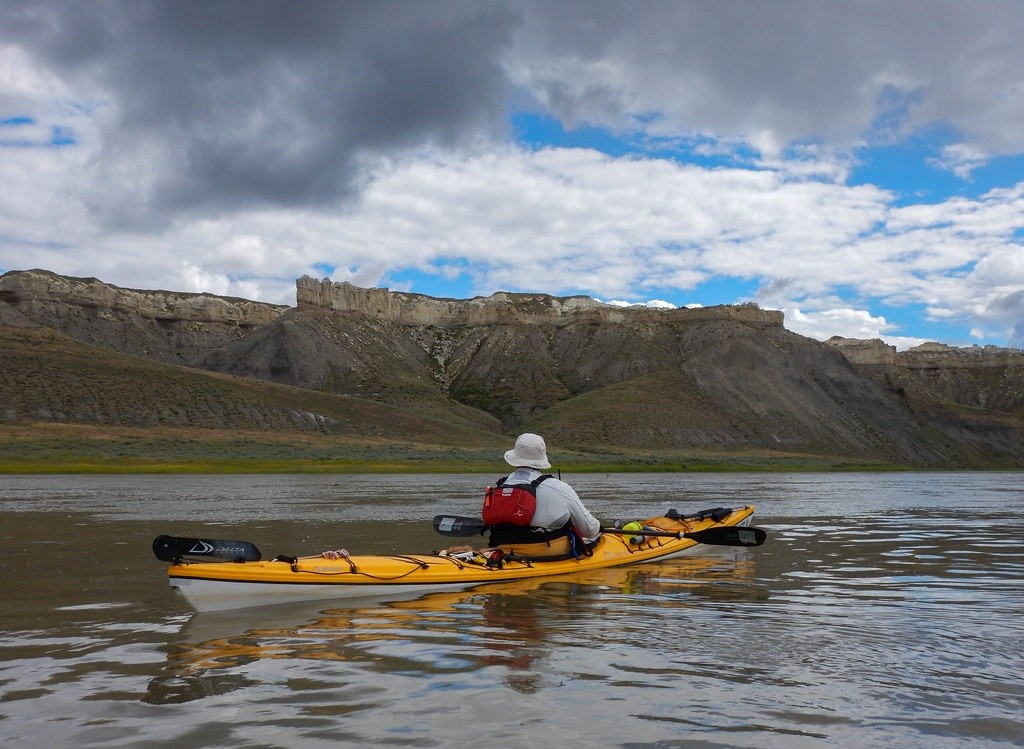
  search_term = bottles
[629,531,656,545]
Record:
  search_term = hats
[504,433,552,470]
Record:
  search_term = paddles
[433,514,767,546]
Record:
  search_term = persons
[482,433,599,558]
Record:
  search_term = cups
[622,522,642,536]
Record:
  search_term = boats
[151,505,754,611]
[146,545,753,704]
